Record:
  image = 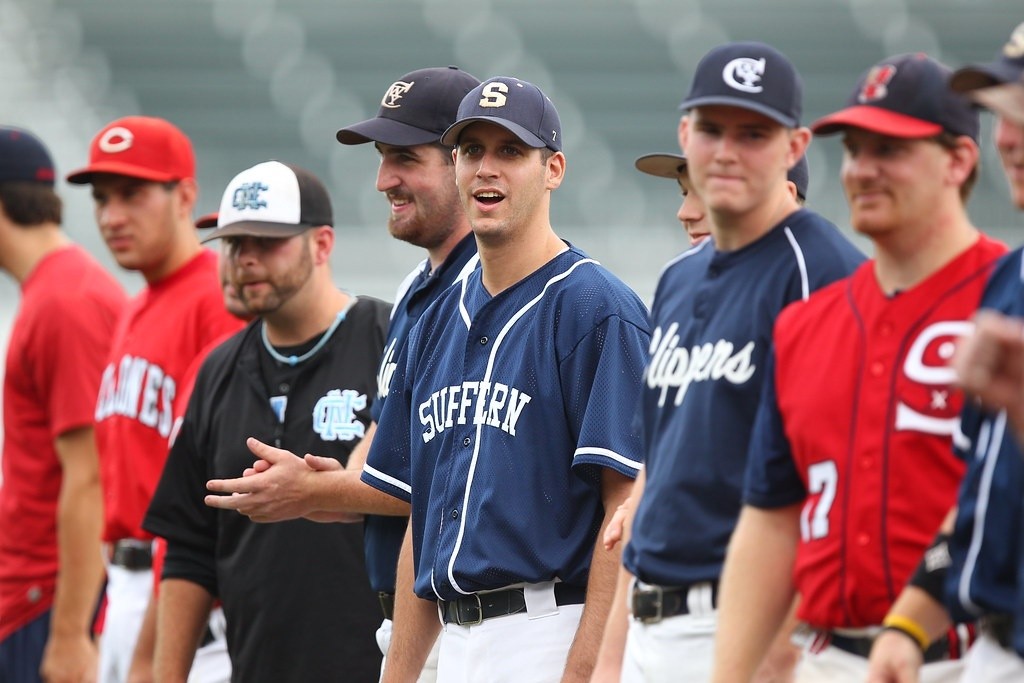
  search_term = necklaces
[262,291,355,365]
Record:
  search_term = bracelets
[879,614,929,652]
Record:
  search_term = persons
[130,159,394,683]
[361,74,649,683]
[709,51,1012,683]
[865,25,1024,683]
[588,48,869,683]
[204,65,483,683]
[66,114,248,683]
[949,307,1024,439]
[0,126,131,683]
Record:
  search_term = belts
[376,593,396,622]
[200,625,216,648]
[434,579,587,627]
[832,629,953,665]
[109,545,154,572]
[629,578,719,626]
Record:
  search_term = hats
[946,21,1023,127]
[439,76,563,151]
[635,121,814,198]
[65,116,197,183]
[0,126,56,183]
[198,160,334,240]
[677,41,803,128]
[334,67,485,145]
[809,54,980,146]
